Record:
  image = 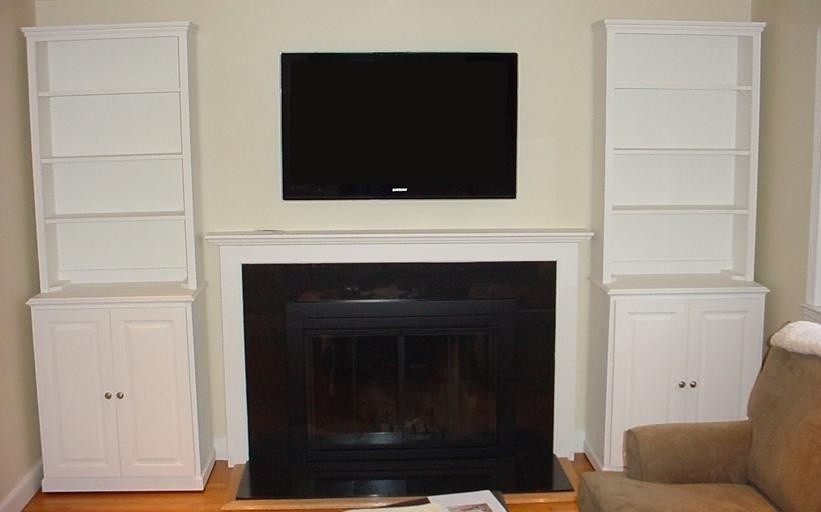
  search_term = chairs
[575,321,821,512]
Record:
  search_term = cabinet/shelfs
[19,22,215,493]
[583,20,771,470]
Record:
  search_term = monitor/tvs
[278,49,519,200]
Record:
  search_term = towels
[769,321,821,358]
